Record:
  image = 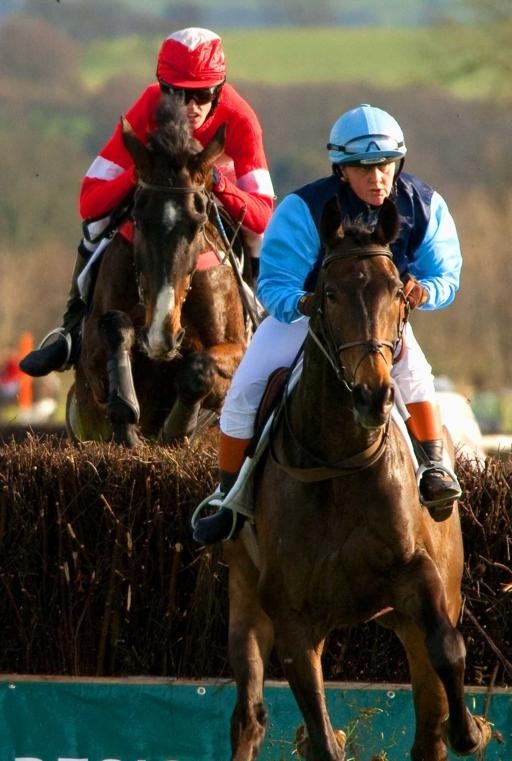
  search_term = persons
[22,27,277,379]
[187,105,462,545]
[463,363,504,435]
[0,328,62,436]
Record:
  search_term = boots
[416,439,459,523]
[195,472,244,540]
[20,242,101,377]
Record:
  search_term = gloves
[404,271,428,310]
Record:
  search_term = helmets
[327,103,407,166]
[158,27,226,90]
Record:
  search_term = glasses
[160,83,215,105]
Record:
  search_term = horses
[68,88,262,450]
[224,192,491,761]
